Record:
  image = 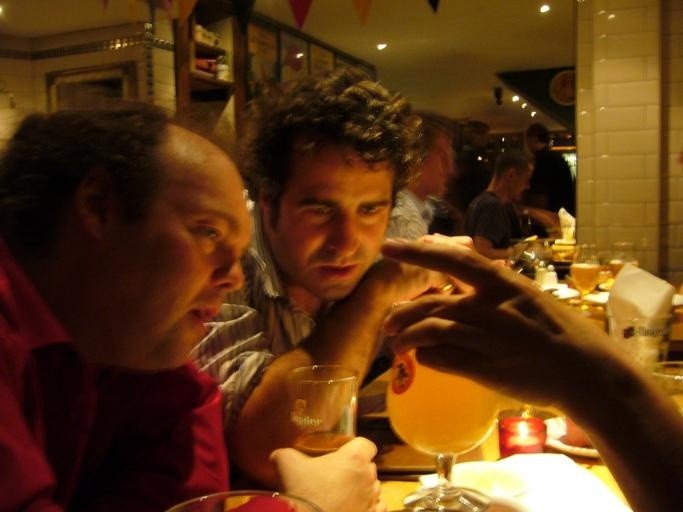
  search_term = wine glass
[570,244,601,313]
[389,302,491,512]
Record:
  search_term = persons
[1,64,683,512]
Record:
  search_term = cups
[500,417,546,460]
[649,362,683,396]
[286,365,358,456]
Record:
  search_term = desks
[211,237,683,509]
